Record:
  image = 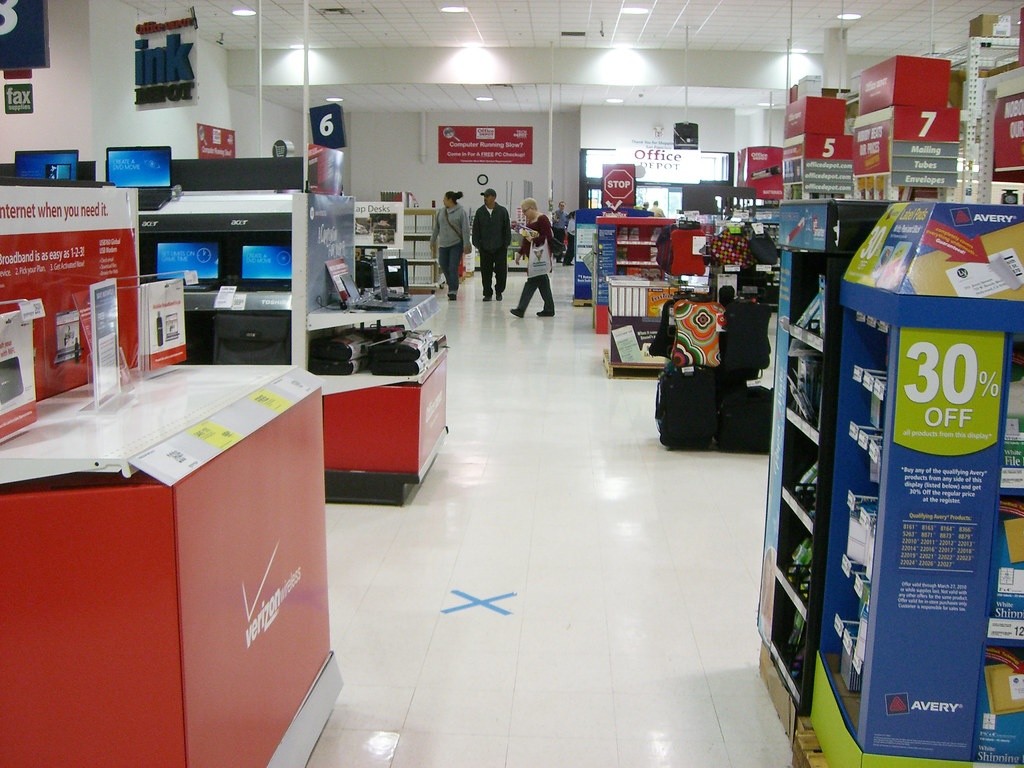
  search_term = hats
[480,189,496,196]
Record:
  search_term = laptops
[235,243,291,292]
[106,146,172,210]
[15,150,79,181]
[155,240,222,292]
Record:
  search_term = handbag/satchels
[526,236,552,278]
[463,240,472,254]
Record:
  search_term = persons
[562,211,575,265]
[430,191,471,300]
[510,198,555,318]
[472,189,511,301]
[651,201,665,217]
[552,201,569,263]
[643,202,649,211]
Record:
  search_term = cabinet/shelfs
[403,208,448,293]
[470,230,530,272]
[594,217,675,334]
[757,197,1024,767]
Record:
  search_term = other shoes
[536,309,552,316]
[556,259,562,263]
[563,261,574,265]
[449,294,456,300]
[496,289,502,301]
[510,309,524,318]
[483,294,492,301]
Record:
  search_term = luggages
[651,290,771,451]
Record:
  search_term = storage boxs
[969,14,1011,38]
[606,274,650,318]
